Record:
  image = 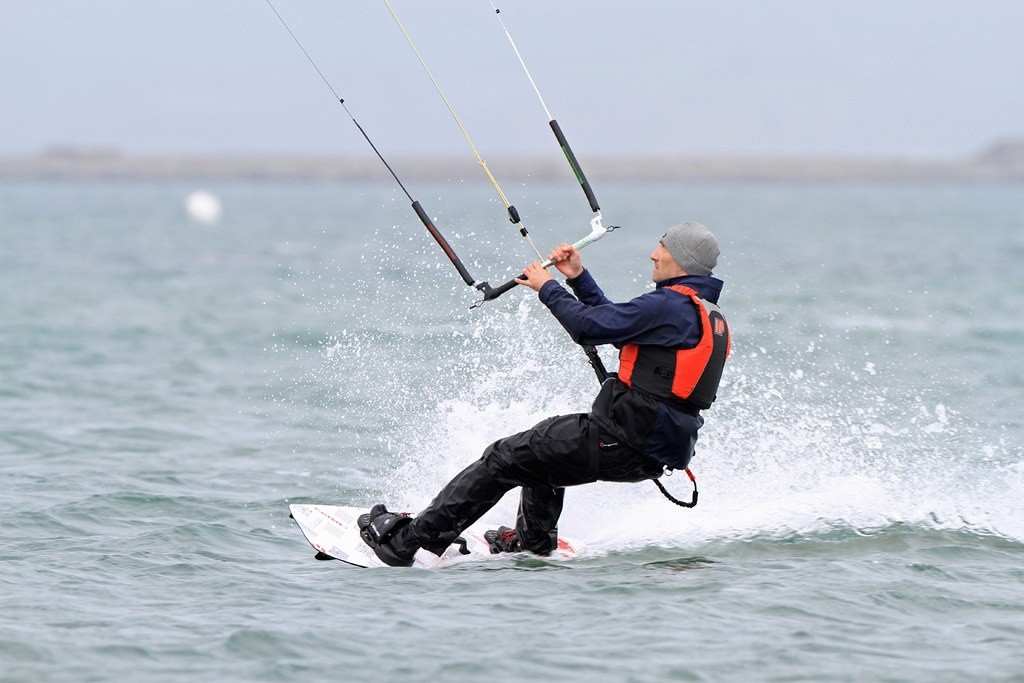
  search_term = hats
[663,221,720,276]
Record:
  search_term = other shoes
[371,504,420,561]
[497,524,547,556]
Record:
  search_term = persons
[356,221,730,569]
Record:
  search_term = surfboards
[288,503,575,569]
